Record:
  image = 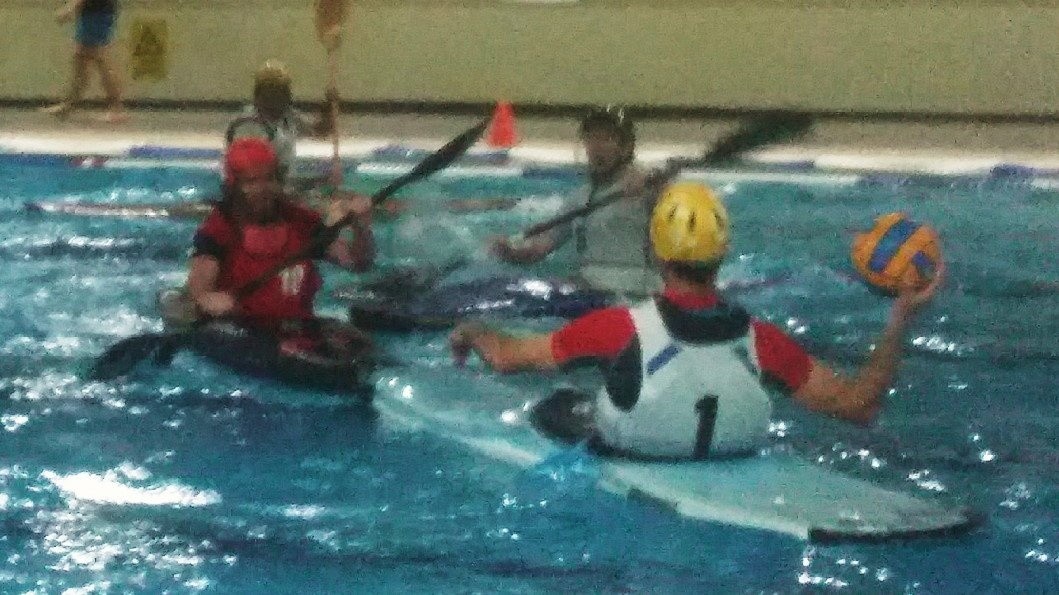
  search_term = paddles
[82,99,498,387]
[310,0,351,202]
[506,107,810,246]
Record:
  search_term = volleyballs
[849,213,940,298]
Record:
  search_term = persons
[183,138,374,342]
[225,57,337,193]
[480,107,679,296]
[448,184,946,459]
[42,0,130,124]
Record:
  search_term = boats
[151,290,402,395]
[367,359,982,561]
[346,252,866,345]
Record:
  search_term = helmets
[226,136,280,185]
[583,102,635,138]
[651,180,730,267]
[256,60,293,88]
